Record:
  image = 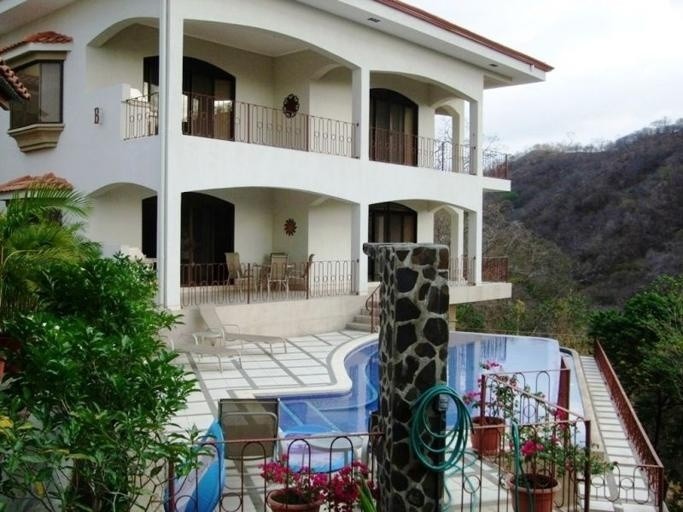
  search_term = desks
[300,432,364,469]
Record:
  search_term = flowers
[458,357,619,485]
[259,453,374,511]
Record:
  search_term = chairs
[158,302,289,373]
[220,249,315,300]
[217,394,287,490]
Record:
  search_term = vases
[265,486,327,511]
[507,472,563,512]
